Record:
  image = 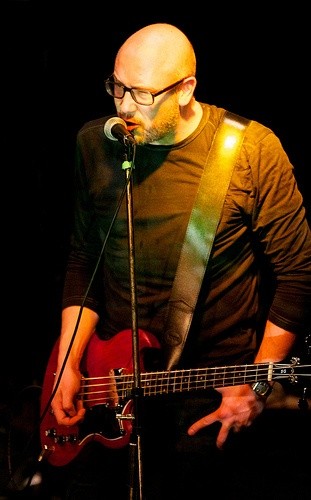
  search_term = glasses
[105,74,191,106]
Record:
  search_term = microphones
[104,117,137,149]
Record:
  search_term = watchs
[297,398,309,410]
[249,379,273,398]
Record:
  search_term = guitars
[38,328,311,477]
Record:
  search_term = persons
[50,23,311,500]
[265,381,311,410]
